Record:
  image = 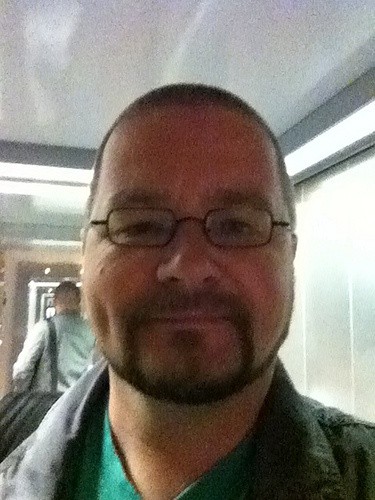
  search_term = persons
[1,84,374,500]
[10,282,102,395]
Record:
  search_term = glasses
[90,205,292,249]
